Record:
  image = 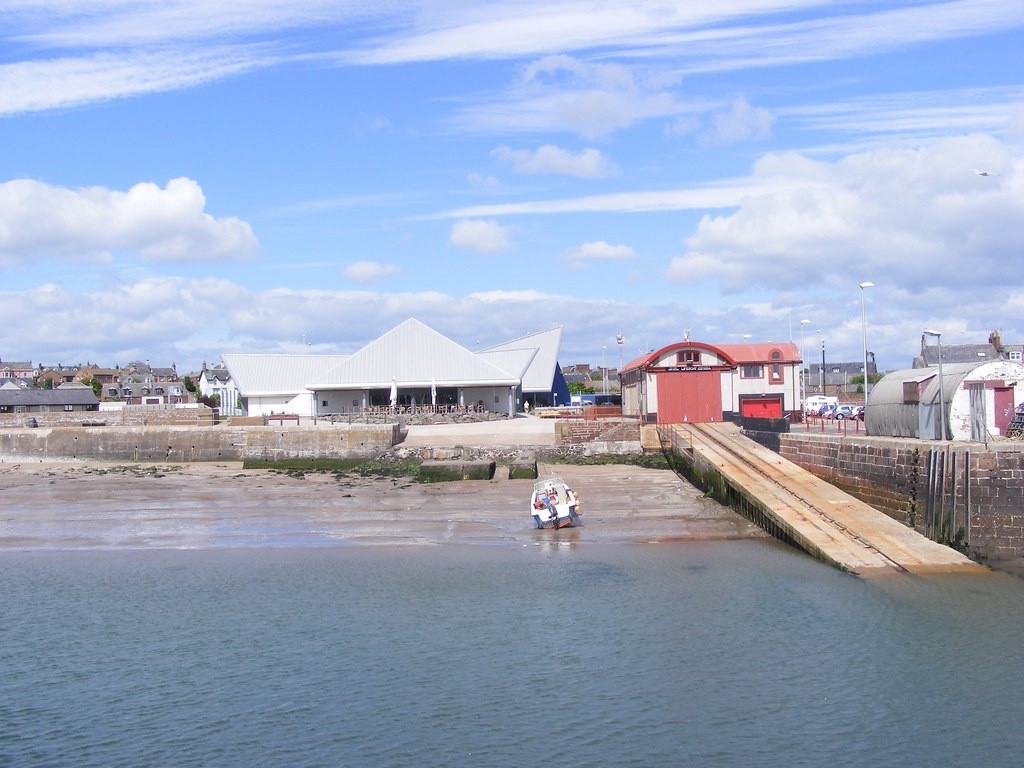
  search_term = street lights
[924,329,947,441]
[800,320,810,424]
[601,346,606,394]
[861,282,874,434]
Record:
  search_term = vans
[834,405,857,420]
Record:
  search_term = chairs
[365,404,483,414]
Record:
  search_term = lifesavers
[548,495,559,506]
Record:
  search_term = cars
[825,407,837,418]
[819,405,834,415]
[852,406,865,420]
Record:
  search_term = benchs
[267,414,299,426]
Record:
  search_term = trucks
[802,395,839,415]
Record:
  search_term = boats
[531,478,582,530]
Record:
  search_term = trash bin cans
[25,416,37,429]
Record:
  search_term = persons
[524,400,530,414]
